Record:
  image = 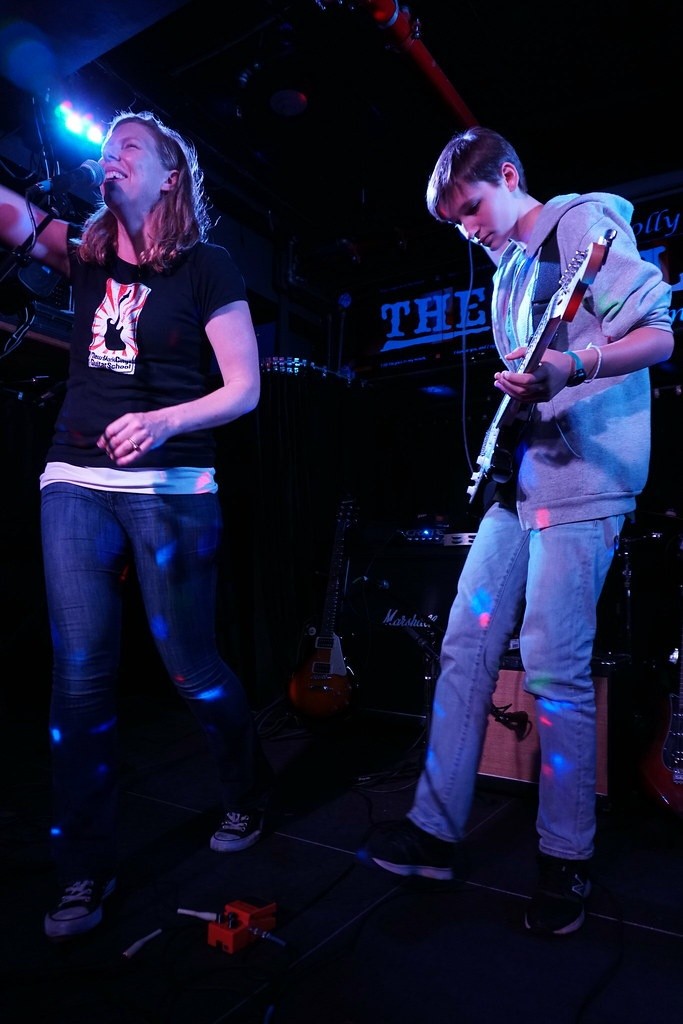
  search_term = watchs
[561,350,586,387]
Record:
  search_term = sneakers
[210,793,271,853]
[45,877,117,937]
[369,825,455,880]
[524,853,592,934]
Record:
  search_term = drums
[591,523,666,668]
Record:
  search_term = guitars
[288,493,362,716]
[638,635,683,813]
[466,228,618,508]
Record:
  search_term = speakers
[422,649,658,804]
[351,544,471,720]
[8,260,65,297]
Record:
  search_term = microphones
[361,576,389,591]
[25,160,106,196]
[495,711,528,723]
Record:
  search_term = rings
[129,439,140,451]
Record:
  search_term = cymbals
[443,532,476,547]
[640,506,683,521]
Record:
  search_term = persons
[0,113,259,938]
[369,128,675,934]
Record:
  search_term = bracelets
[583,342,602,384]
